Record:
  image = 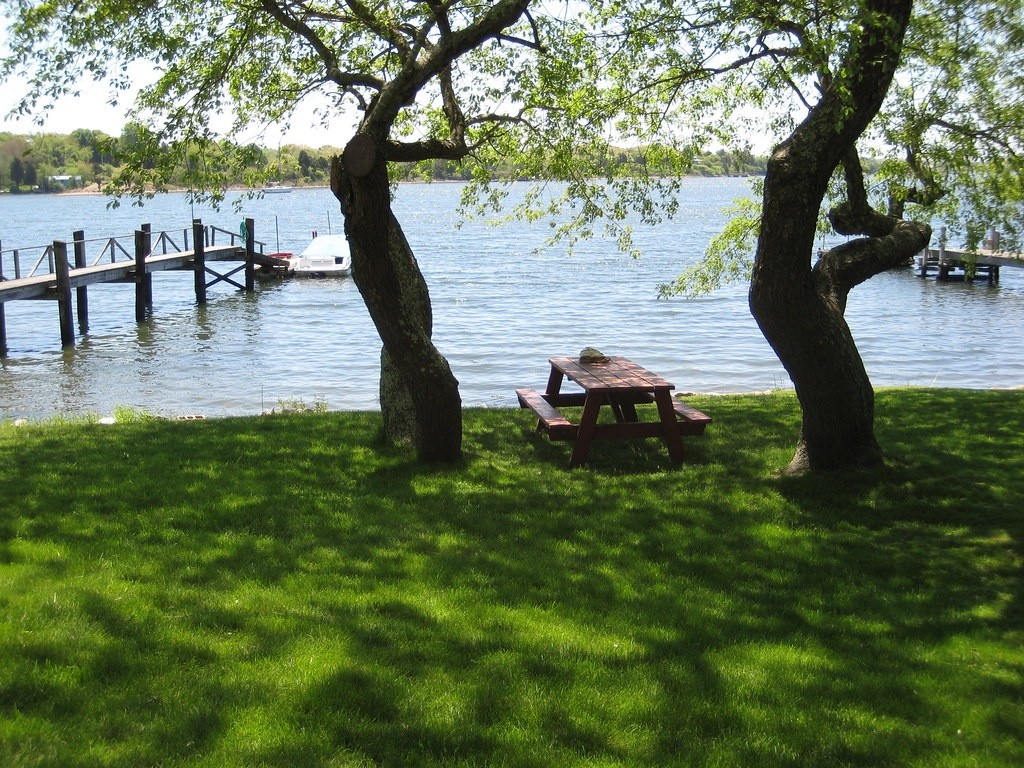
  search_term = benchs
[515,388,572,429]
[649,392,712,423]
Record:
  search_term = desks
[518,356,706,474]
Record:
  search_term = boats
[261,184,292,194]
[294,211,352,278]
[253,216,298,278]
